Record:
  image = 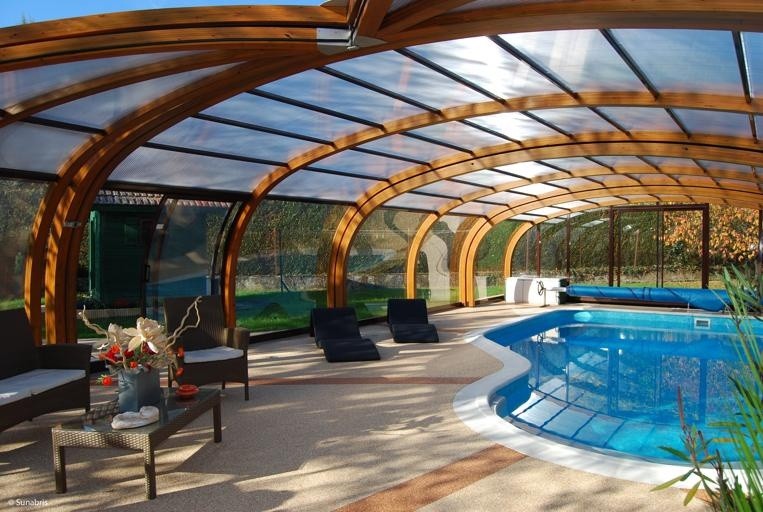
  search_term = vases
[118,369,160,413]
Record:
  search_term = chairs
[163,294,251,400]
[310,308,380,362]
[387,299,439,342]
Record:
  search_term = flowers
[78,296,204,386]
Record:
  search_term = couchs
[0,308,92,433]
